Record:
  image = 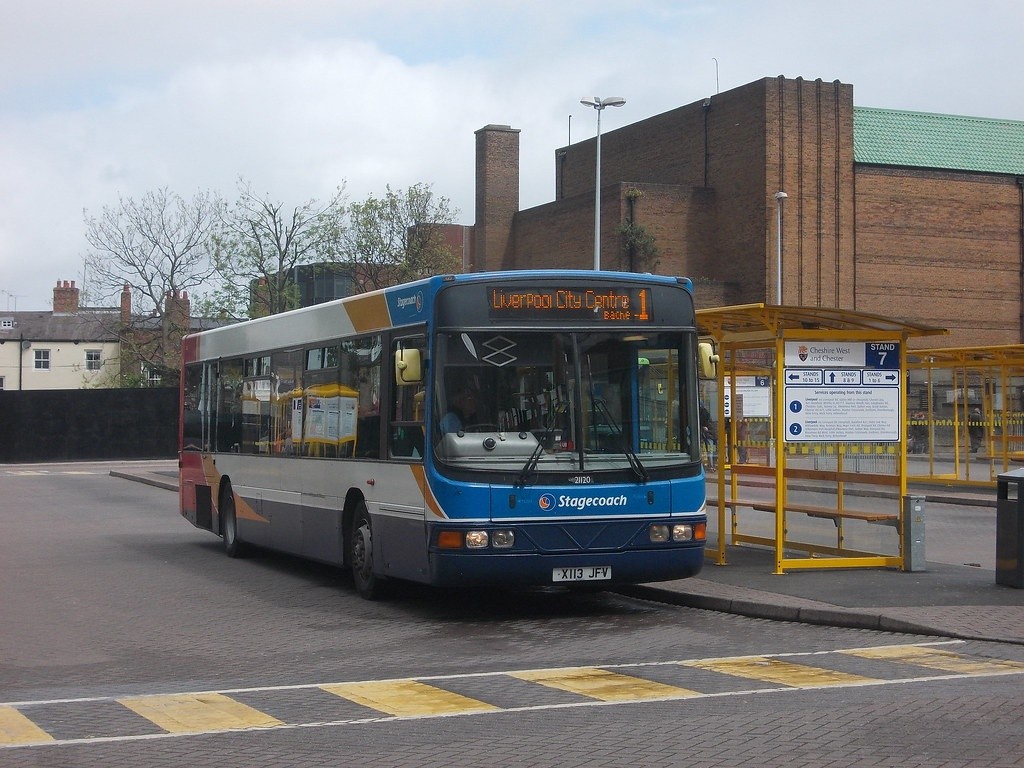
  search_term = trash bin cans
[996,466,1024,588]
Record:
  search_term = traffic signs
[782,367,902,444]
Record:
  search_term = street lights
[579,95,625,271]
[775,191,788,305]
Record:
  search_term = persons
[909,411,929,454]
[439,371,497,433]
[969,408,983,452]
[284,429,292,455]
[673,400,715,472]
[725,417,748,464]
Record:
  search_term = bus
[177,265,722,602]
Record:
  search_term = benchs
[975,435,1024,461]
[705,465,902,536]
